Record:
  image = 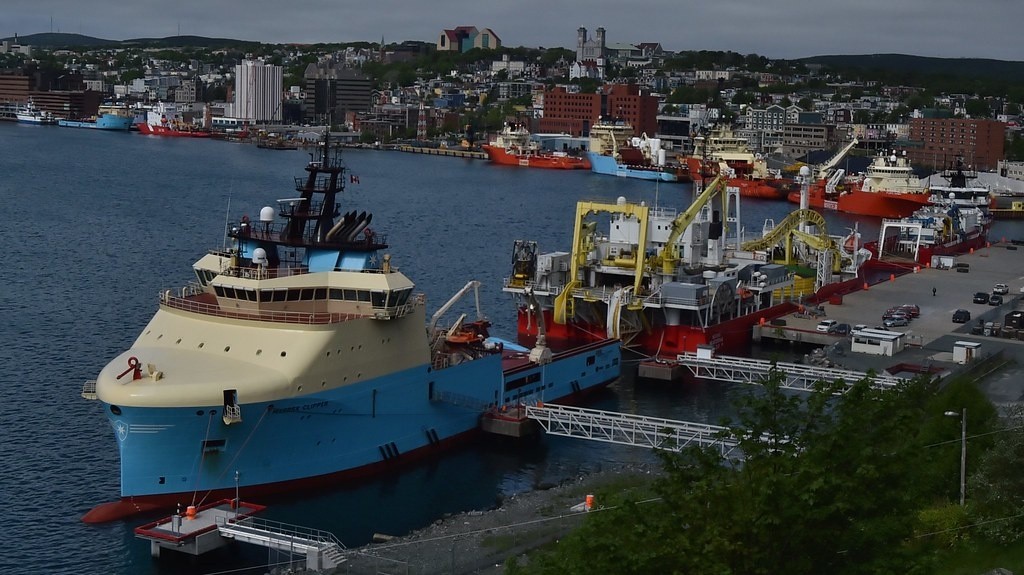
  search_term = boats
[685,158,780,198]
[58,114,136,130]
[481,145,592,169]
[586,150,678,181]
[135,122,252,142]
[255,128,300,150]
[864,203,993,269]
[500,188,916,360]
[81,124,622,525]
[788,147,935,220]
[15,95,66,125]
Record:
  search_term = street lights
[944,408,968,506]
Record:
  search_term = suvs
[992,283,1010,295]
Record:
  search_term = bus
[930,184,991,206]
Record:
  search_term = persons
[932,287,936,296]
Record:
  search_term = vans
[816,320,839,332]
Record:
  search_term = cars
[852,324,867,335]
[952,308,970,323]
[832,322,852,334]
[972,291,990,304]
[881,303,921,326]
[989,294,1003,306]
